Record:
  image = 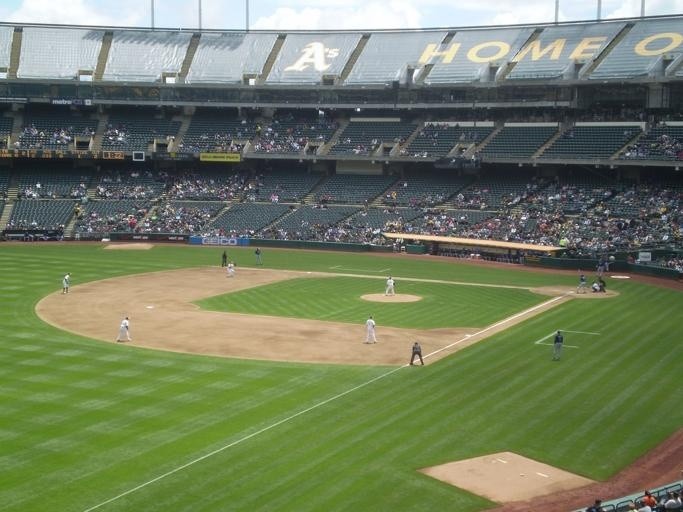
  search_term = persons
[551,331,563,360]
[585,499,606,511]
[591,281,599,293]
[408,342,424,366]
[576,274,586,294]
[384,276,396,296]
[115,316,132,342]
[598,277,606,293]
[364,316,377,344]
[62,272,71,293]
[625,489,683,512]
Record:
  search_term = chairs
[383,112,683,277]
[0,110,395,243]
